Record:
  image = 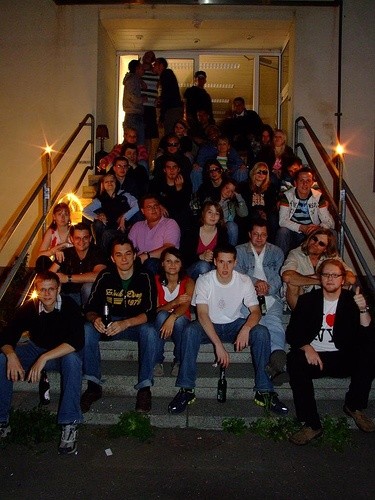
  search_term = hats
[194,71,206,79]
[129,58,141,73]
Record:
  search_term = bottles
[102,302,113,341]
[217,366,227,403]
[39,367,50,406]
[257,294,266,316]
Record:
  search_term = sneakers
[291,425,322,445]
[272,371,290,387]
[80,381,102,413]
[168,388,196,416]
[254,391,289,416]
[58,423,79,455]
[135,389,152,413]
[342,404,375,431]
[0,420,11,439]
[265,350,287,376]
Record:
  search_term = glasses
[146,204,158,210]
[128,135,136,138]
[257,170,267,175]
[312,236,327,247]
[41,288,55,293]
[117,165,128,169]
[167,143,177,147]
[252,232,268,238]
[209,168,219,173]
[322,273,343,278]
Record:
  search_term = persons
[154,247,195,376]
[183,71,215,125]
[79,235,159,413]
[276,167,335,256]
[153,58,182,133]
[82,173,139,242]
[138,50,160,139]
[235,217,288,379]
[100,97,322,244]
[182,200,229,280]
[123,60,148,145]
[0,271,85,455]
[285,259,375,446]
[168,242,289,416]
[128,195,180,276]
[281,227,355,312]
[35,203,74,273]
[49,223,108,308]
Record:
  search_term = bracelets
[173,313,178,318]
[56,261,62,266]
[360,309,367,313]
[68,274,72,282]
[142,251,150,259]
